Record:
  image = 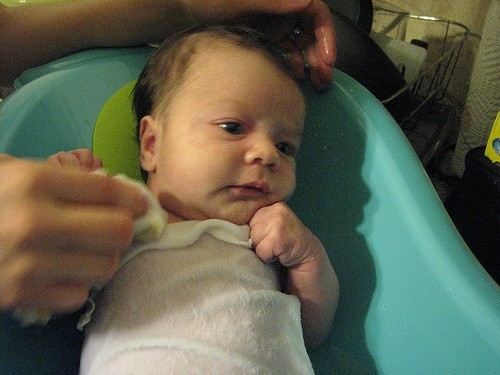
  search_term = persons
[0,0,336,314]
[44,27,340,374]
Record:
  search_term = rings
[290,22,299,39]
